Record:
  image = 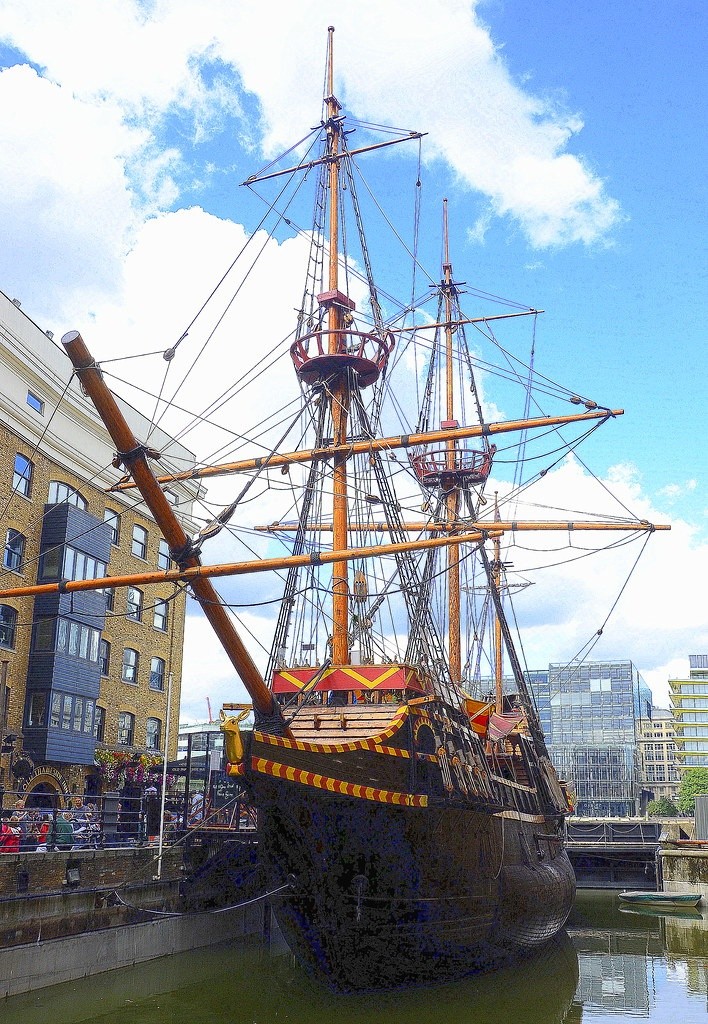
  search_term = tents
[148,753,229,780]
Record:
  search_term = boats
[617,902,704,922]
[615,889,705,907]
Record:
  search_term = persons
[1,782,204,851]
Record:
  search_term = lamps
[2,734,17,754]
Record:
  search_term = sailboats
[0,27,672,1001]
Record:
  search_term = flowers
[94,748,177,789]
